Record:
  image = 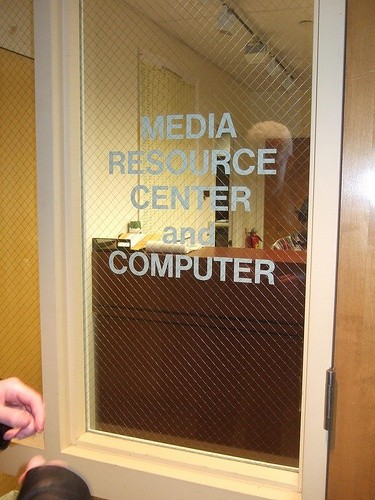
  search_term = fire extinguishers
[247,228,263,250]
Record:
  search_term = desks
[93,239,307,458]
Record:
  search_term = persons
[1,377,93,500]
[270,199,308,286]
[243,120,295,196]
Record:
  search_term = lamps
[247,41,269,64]
[277,73,296,92]
[265,56,282,78]
[219,5,235,36]
